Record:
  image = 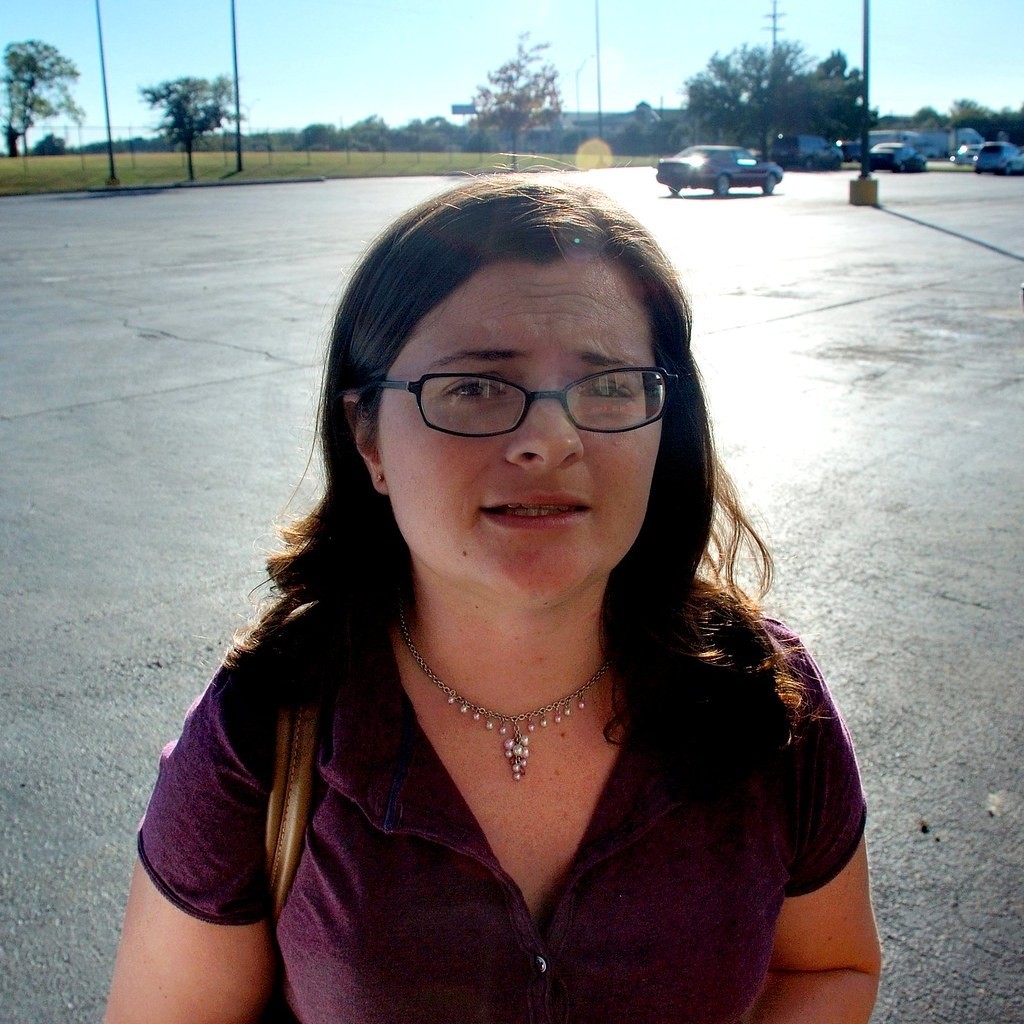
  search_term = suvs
[772,135,845,171]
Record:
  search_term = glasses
[377,365,680,438]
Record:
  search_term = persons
[101,180,888,1024]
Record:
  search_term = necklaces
[395,593,614,784]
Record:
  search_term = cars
[655,144,783,198]
[949,141,1024,176]
[868,143,929,172]
